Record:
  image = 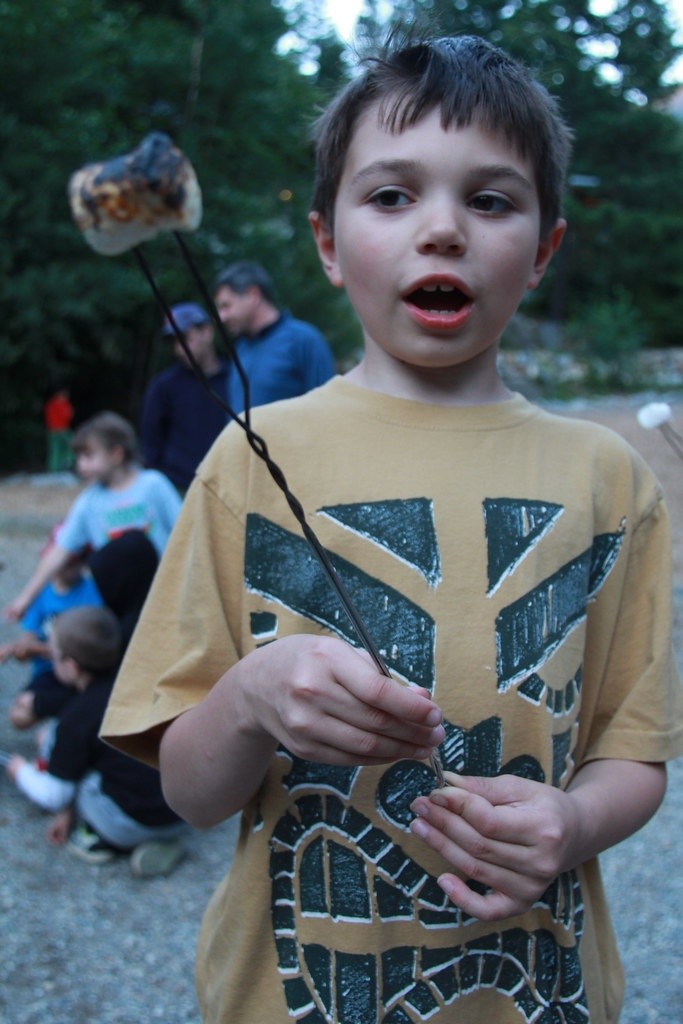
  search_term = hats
[164,303,211,335]
[41,520,91,566]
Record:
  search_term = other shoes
[131,839,185,878]
[66,825,115,865]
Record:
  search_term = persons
[92,18,683,1024]
[0,258,338,876]
